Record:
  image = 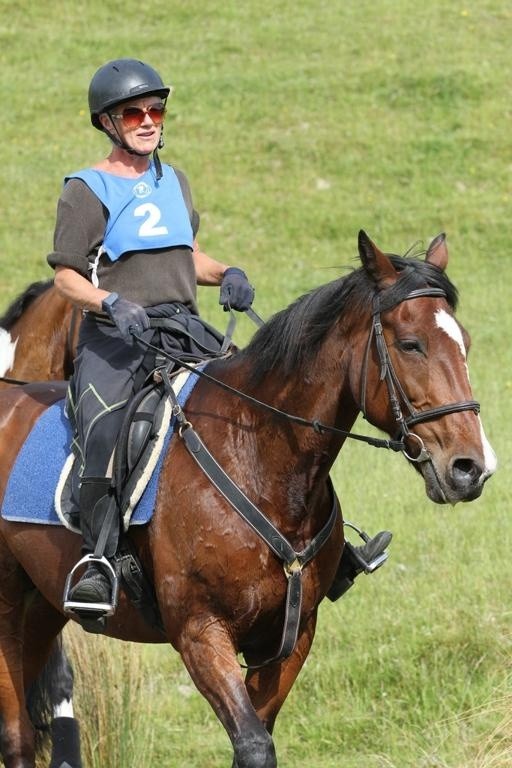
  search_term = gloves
[219,267,255,312]
[102,291,151,348]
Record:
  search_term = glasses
[109,103,166,128]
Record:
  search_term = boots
[69,475,115,634]
[326,530,393,602]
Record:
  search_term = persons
[46,58,393,633]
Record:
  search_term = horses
[0,278,90,391]
[0,228,499,768]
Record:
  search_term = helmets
[88,59,170,131]
